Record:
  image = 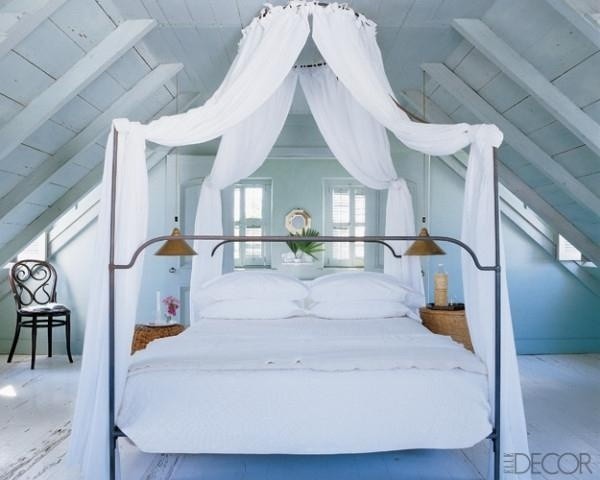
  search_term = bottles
[434,264,448,307]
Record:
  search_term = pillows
[197,268,422,322]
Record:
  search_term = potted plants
[287,227,326,263]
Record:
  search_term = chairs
[6,259,73,369]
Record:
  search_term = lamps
[155,73,197,255]
[404,69,443,256]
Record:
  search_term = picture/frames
[285,210,311,236]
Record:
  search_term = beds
[113,235,501,480]
